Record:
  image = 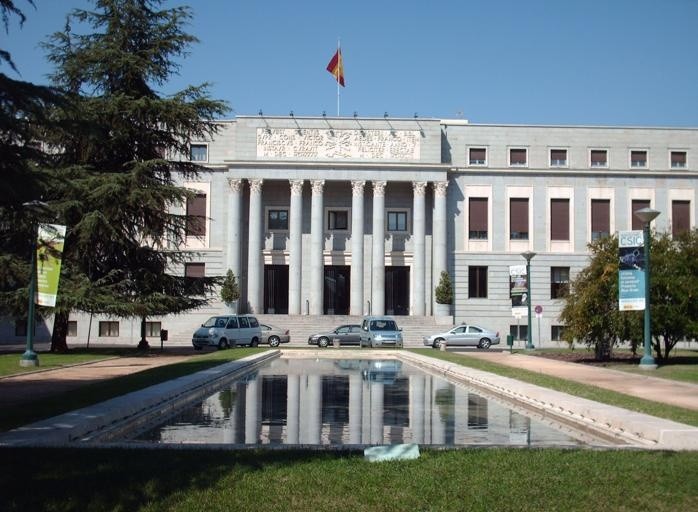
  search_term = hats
[260,324,290,347]
[308,325,360,347]
[423,325,500,349]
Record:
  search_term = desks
[634,207,661,364]
[20,199,50,368]
[520,250,536,349]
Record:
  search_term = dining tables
[220,387,238,420]
[435,377,454,423]
[221,269,241,315]
[434,270,452,316]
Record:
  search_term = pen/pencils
[192,316,262,350]
[360,316,403,348]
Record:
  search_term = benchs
[326,49,344,87]
